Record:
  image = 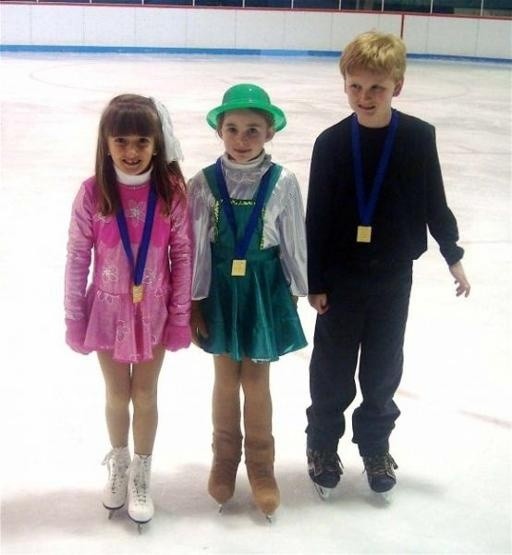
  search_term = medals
[133,286,143,303]
[231,260,247,277]
[356,226,372,243]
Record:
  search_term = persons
[63,94,196,524]
[187,83,307,517]
[305,29,471,493]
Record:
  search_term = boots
[101,447,131,510]
[208,433,241,502]
[246,446,279,513]
[127,453,154,523]
[306,445,342,488]
[363,451,397,493]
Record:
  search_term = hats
[207,84,287,132]
